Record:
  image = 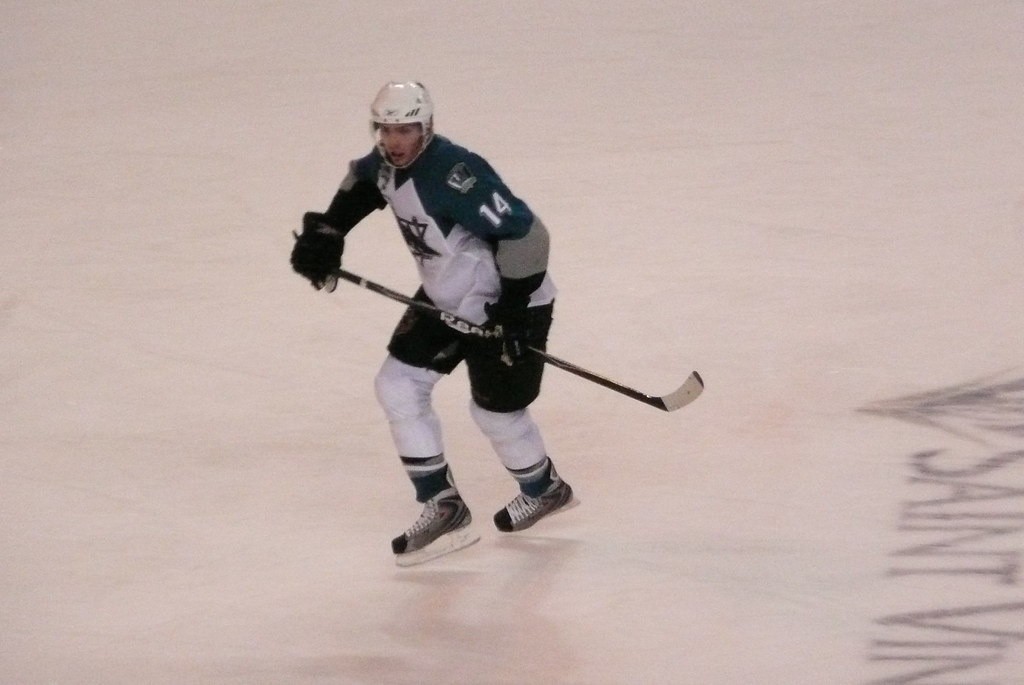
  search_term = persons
[290,81,581,567]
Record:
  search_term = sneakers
[494,456,573,532]
[391,487,480,565]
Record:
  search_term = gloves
[290,212,343,292]
[481,302,529,369]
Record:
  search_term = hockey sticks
[337,269,706,414]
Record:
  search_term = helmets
[367,80,433,147]
